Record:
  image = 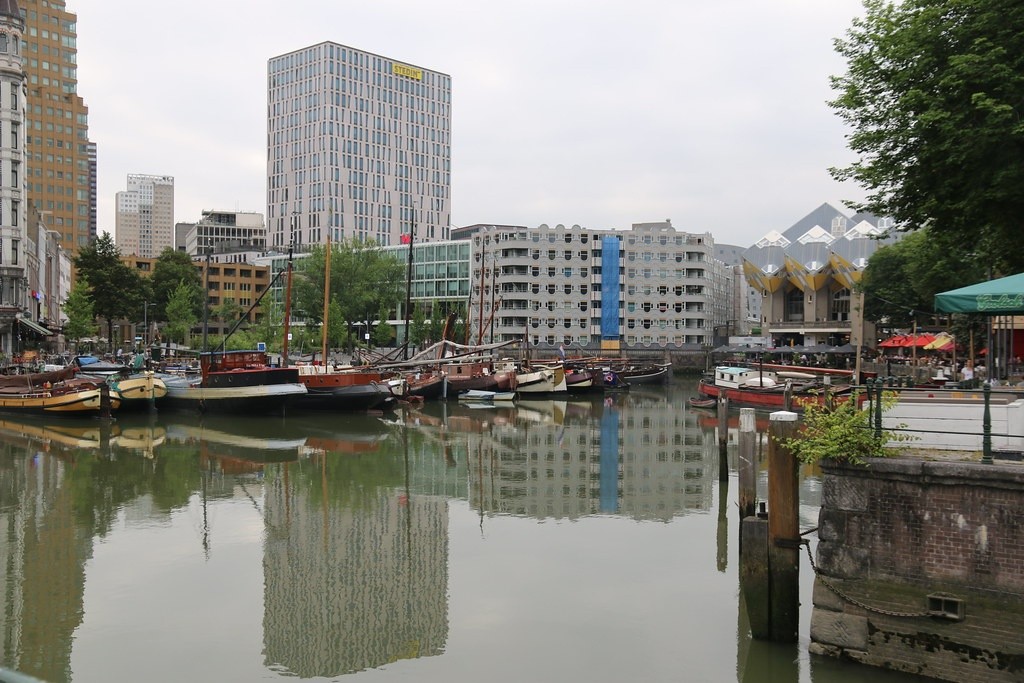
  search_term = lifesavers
[606,376,612,382]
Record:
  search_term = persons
[70,344,144,362]
[960,359,974,380]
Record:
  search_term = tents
[934,271,1024,392]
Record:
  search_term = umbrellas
[877,332,962,358]
[708,343,871,369]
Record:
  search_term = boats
[699,364,870,410]
[689,396,716,409]
[0,203,671,418]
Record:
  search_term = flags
[402,232,410,244]
